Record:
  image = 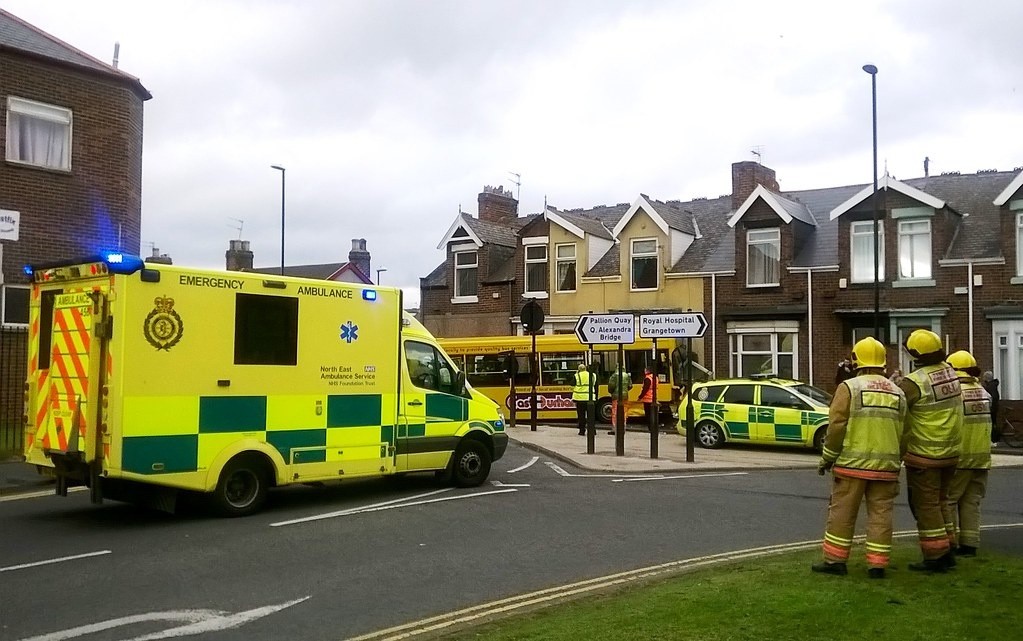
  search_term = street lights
[861,63,880,341]
[377,268,388,285]
[270,165,284,276]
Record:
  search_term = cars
[675,373,836,455]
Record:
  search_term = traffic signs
[574,315,635,344]
[639,312,708,339]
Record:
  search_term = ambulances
[23,251,509,518]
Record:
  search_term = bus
[434,330,676,424]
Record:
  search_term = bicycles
[997,405,1023,448]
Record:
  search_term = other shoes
[812,562,849,575]
[907,551,956,572]
[869,569,885,578]
[958,546,977,555]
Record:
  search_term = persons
[889,369,902,385]
[570,364,599,436]
[946,350,993,557]
[834,357,856,387]
[982,370,999,449]
[812,337,907,578]
[607,363,633,435]
[637,366,659,433]
[898,329,964,572]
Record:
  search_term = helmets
[852,337,887,370]
[946,350,980,378]
[902,329,946,363]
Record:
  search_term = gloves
[818,457,833,476]
